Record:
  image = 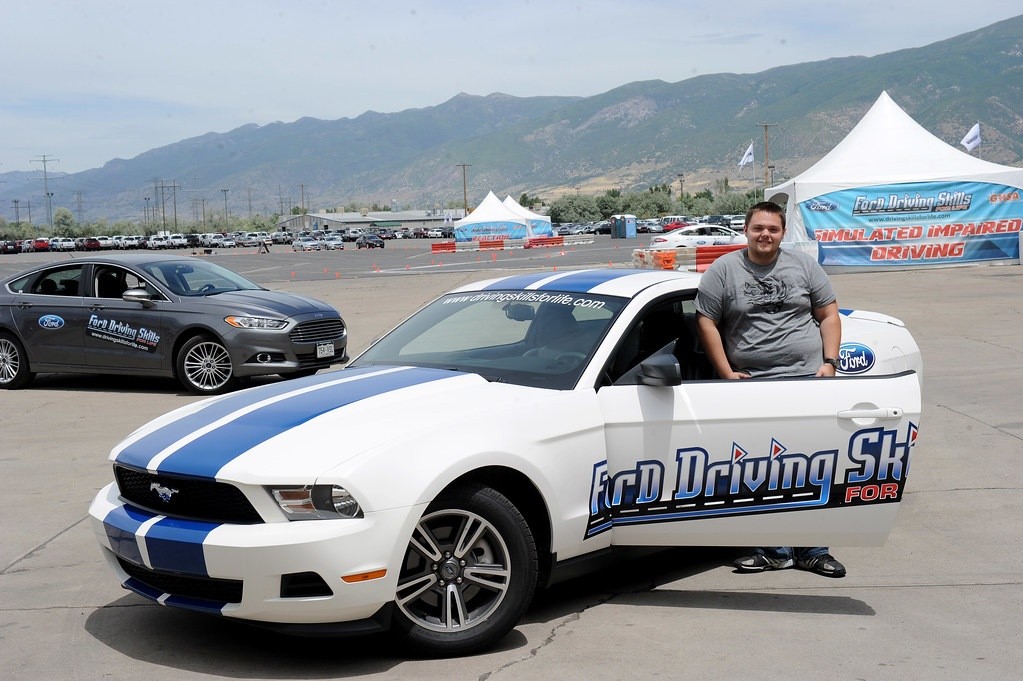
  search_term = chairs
[602,299,694,388]
[98,273,122,298]
[523,308,582,360]
[41,279,64,296]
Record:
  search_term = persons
[692,202,847,578]
[76,239,91,251]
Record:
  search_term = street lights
[221,189,229,233]
[768,166,775,187]
[144,197,151,235]
[46,193,55,238]
[456,164,472,217]
[677,174,686,214]
[755,123,778,187]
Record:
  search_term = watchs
[824,358,841,370]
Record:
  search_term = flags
[960,123,981,153]
[737,141,754,172]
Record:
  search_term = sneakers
[795,547,847,578]
[733,552,794,572]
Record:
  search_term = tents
[454,190,552,242]
[764,90,1023,267]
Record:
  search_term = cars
[0,227,455,253]
[88,265,926,658]
[636,215,747,233]
[551,221,611,235]
[0,254,350,396]
[291,237,321,252]
[355,235,384,249]
[316,237,345,251]
